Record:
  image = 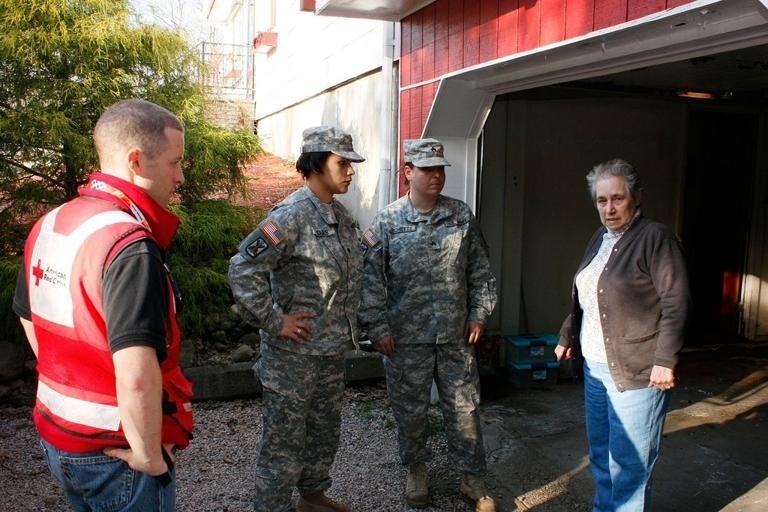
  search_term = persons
[361,139,501,511]
[13,100,197,510]
[554,160,690,511]
[226,126,363,511]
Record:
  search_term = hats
[403,137,452,168]
[299,125,366,163]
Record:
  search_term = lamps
[673,87,736,101]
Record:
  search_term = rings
[296,327,302,334]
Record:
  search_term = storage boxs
[504,332,558,364]
[505,360,560,390]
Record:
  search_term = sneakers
[296,489,350,512]
[458,472,497,512]
[405,460,430,508]
